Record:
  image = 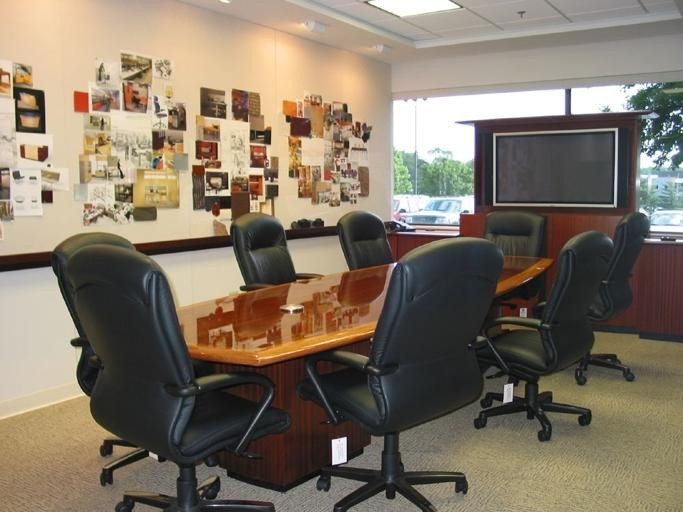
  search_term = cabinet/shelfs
[386,231,460,263]
[639,239,683,342]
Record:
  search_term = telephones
[387,221,416,231]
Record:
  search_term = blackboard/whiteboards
[0,0,393,273]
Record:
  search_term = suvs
[400,198,474,225]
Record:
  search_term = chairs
[231,212,323,291]
[483,209,547,321]
[533,212,650,386]
[298,238,504,512]
[66,244,292,512]
[51,232,149,486]
[337,211,394,272]
[474,232,614,441]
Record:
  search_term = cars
[645,211,682,233]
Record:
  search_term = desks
[175,257,557,491]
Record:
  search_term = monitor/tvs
[491,128,627,209]
